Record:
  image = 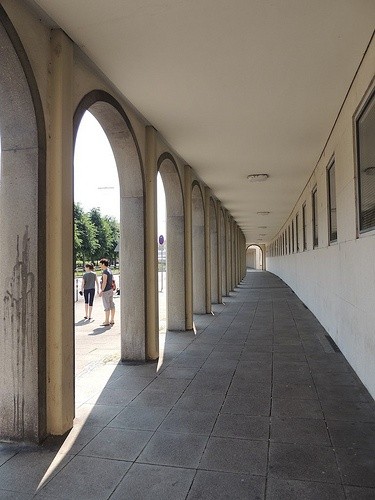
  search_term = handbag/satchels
[112,279,116,290]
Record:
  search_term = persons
[98,259,115,326]
[80,263,99,321]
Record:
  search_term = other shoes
[84,316,91,320]
[100,320,115,326]
